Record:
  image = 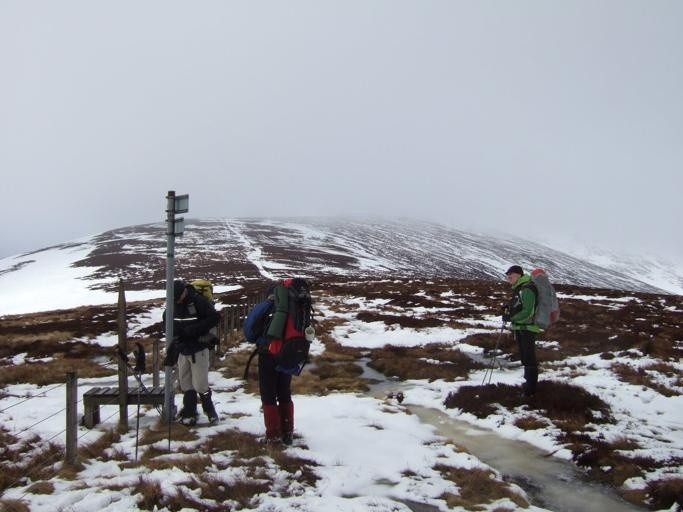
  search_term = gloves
[131,341,147,373]
[500,313,511,322]
[500,301,510,312]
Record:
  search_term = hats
[504,265,524,277]
[174,279,186,301]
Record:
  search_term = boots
[198,388,220,425]
[175,389,199,419]
[257,400,283,448]
[278,399,294,446]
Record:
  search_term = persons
[242,282,295,445]
[161,279,223,427]
[497,265,539,392]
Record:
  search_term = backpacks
[188,279,218,350]
[520,267,560,330]
[267,276,314,369]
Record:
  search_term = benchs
[81,384,176,423]
